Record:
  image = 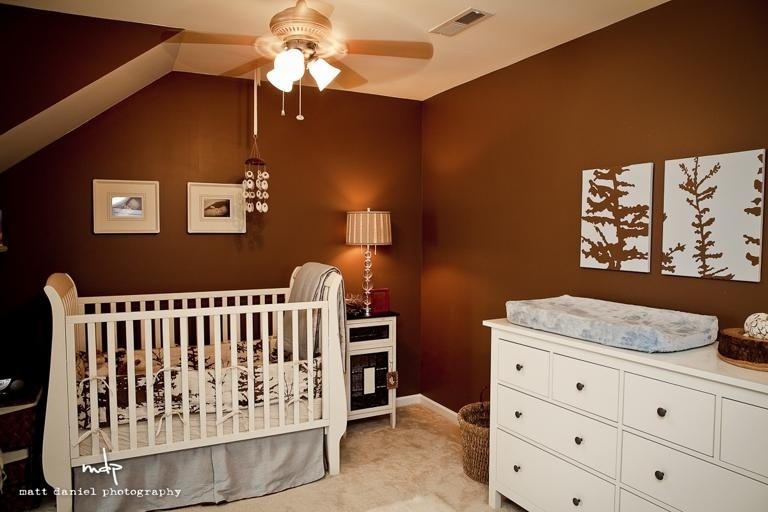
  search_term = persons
[113,197,142,210]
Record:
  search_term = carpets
[369,494,456,511]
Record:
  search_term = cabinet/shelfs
[482,318,768,512]
[342,311,399,439]
[0,385,42,512]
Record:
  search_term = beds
[43,262,348,512]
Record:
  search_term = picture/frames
[187,180,247,233]
[367,288,390,314]
[92,177,161,234]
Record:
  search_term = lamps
[346,208,392,318]
[266,46,340,121]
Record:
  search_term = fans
[163,1,433,89]
